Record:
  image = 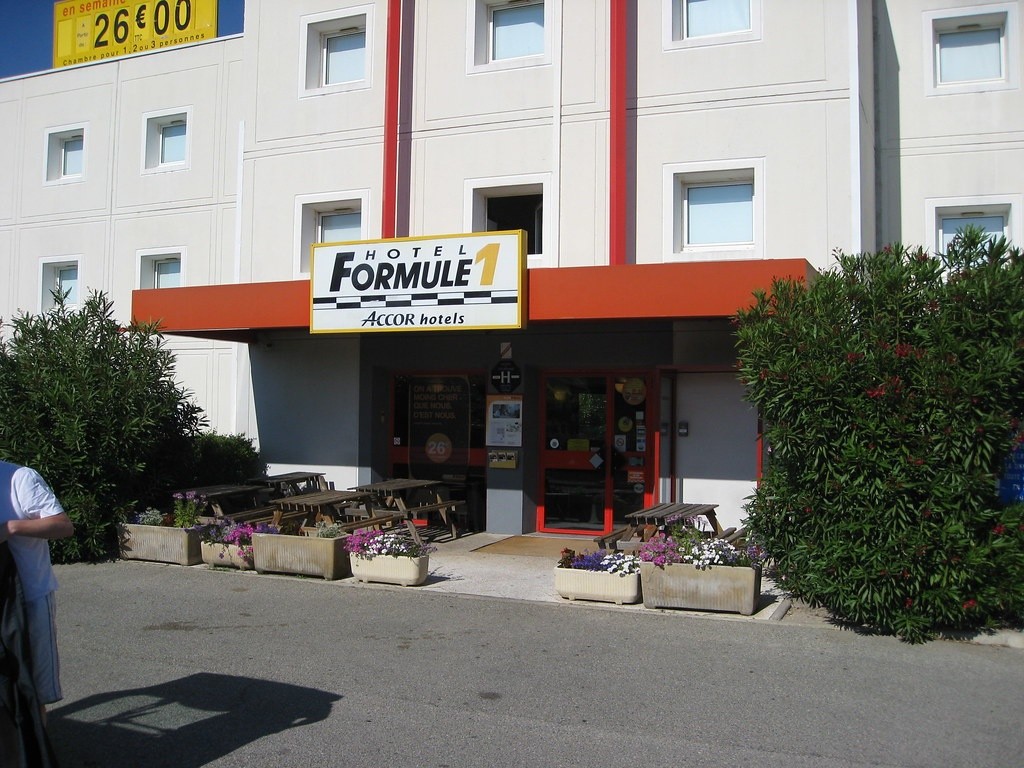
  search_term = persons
[0,461,75,729]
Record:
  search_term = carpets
[470,535,600,558]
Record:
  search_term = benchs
[593,527,629,550]
[225,500,466,533]
[714,527,747,543]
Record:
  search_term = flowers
[557,547,641,577]
[253,520,347,539]
[126,491,208,528]
[639,512,767,571]
[184,518,256,561]
[342,529,438,567]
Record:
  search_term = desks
[246,472,329,496]
[614,502,724,554]
[268,490,383,533]
[346,478,459,544]
[169,483,275,525]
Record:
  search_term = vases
[116,522,203,566]
[639,562,761,616]
[554,564,641,605]
[200,541,255,571]
[350,553,429,587]
[252,534,352,581]
